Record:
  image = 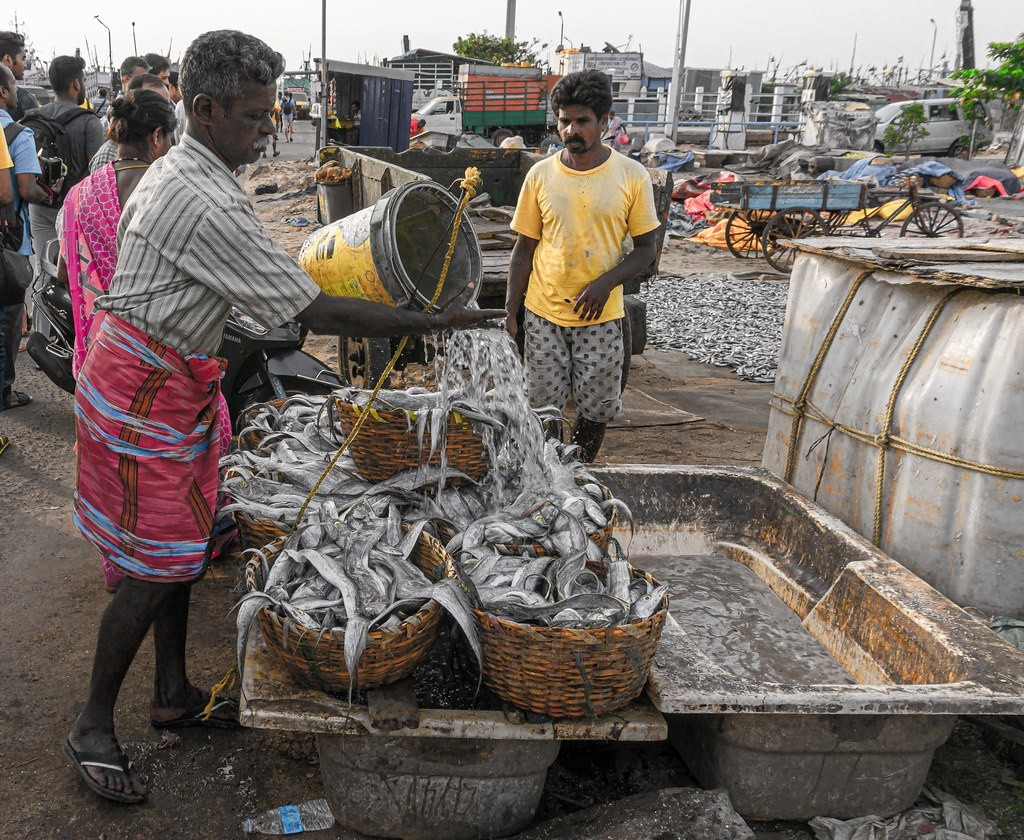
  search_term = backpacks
[283,99,292,115]
[0,121,26,249]
[14,107,100,208]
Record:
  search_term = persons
[66,30,509,804]
[600,111,627,152]
[276,91,298,143]
[0,31,187,459]
[345,101,362,145]
[261,100,281,158]
[409,118,426,136]
[499,70,661,464]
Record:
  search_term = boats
[9,10,45,85]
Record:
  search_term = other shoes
[273,151,280,157]
[285,137,293,143]
[263,154,267,158]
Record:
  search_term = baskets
[236,395,327,457]
[436,477,616,557]
[246,525,456,693]
[458,560,667,718]
[227,470,292,563]
[335,397,491,496]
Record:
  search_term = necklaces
[112,157,150,171]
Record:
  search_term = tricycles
[710,172,964,273]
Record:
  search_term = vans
[874,98,994,159]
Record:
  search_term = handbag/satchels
[0,240,34,305]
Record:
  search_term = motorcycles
[26,237,348,435]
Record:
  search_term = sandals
[0,435,11,455]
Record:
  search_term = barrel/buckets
[298,180,483,313]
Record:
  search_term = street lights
[558,11,564,75]
[132,22,138,57]
[929,19,937,78]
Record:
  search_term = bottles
[242,799,334,834]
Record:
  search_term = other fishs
[627,276,790,383]
[213,383,669,709]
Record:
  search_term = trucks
[410,79,548,148]
[284,79,312,120]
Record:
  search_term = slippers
[0,390,32,412]
[150,691,247,730]
[63,737,149,804]
